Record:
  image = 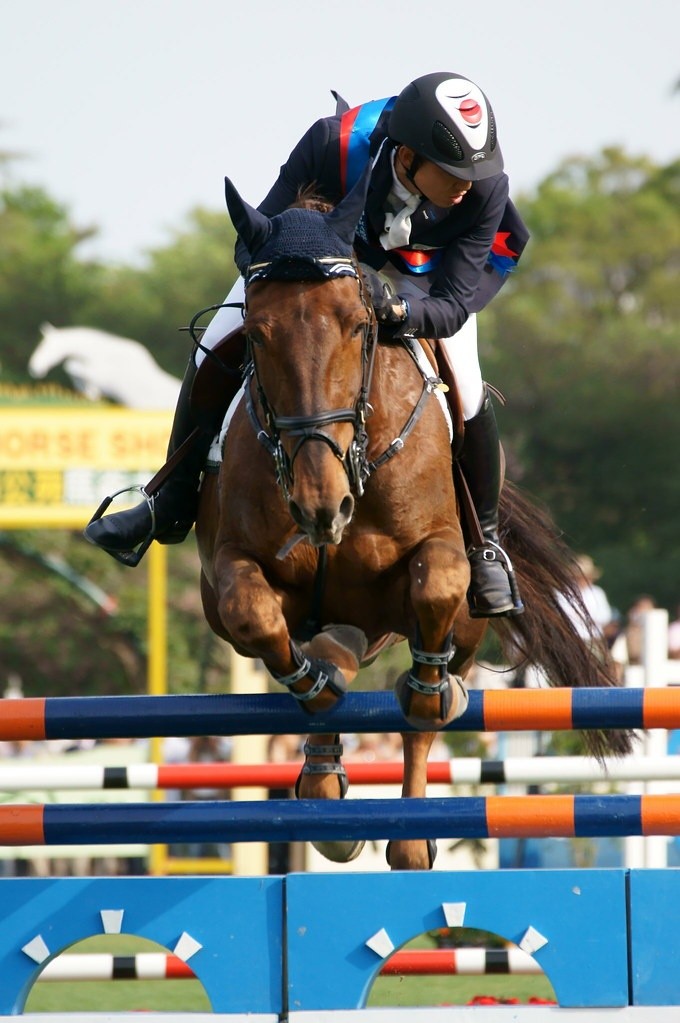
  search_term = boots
[82,332,213,549]
[453,381,515,614]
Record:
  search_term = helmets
[388,72,504,181]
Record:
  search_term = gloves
[359,263,406,323]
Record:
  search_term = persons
[159,729,481,875]
[85,72,529,617]
[559,554,651,664]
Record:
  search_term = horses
[191,160,649,874]
